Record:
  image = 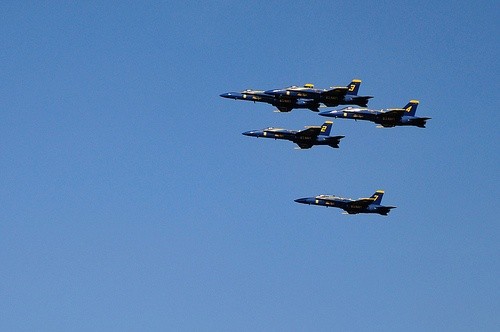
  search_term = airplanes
[293,190,397,216]
[218,78,374,113]
[318,100,432,130]
[241,121,345,151]
